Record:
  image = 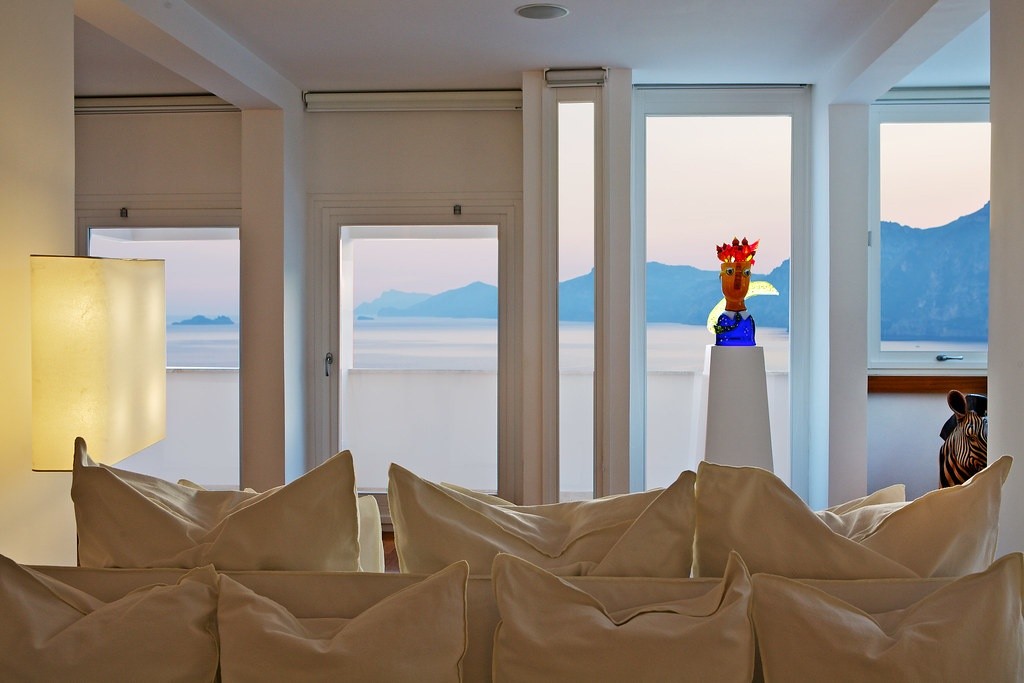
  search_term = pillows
[0,436,1023,683]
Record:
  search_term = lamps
[30,254,165,473]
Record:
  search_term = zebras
[938,389,987,490]
